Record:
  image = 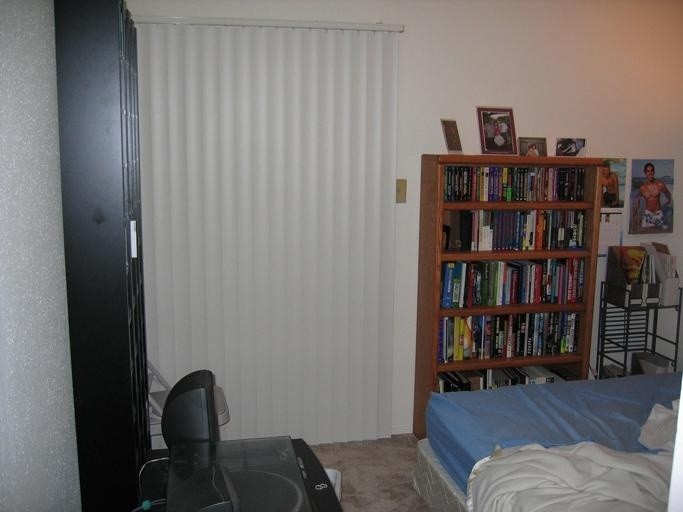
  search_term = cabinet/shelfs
[410,153,603,441]
[54,0,151,512]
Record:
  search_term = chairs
[131,368,237,512]
[144,359,240,512]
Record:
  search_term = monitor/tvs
[161,370,217,449]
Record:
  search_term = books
[434,165,586,394]
[619,240,677,282]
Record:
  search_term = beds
[408,372,683,511]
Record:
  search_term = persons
[601,162,619,206]
[527,145,533,153]
[525,145,538,155]
[492,120,505,147]
[631,162,671,230]
[498,117,508,148]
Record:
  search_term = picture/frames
[475,106,519,155]
[517,136,547,158]
